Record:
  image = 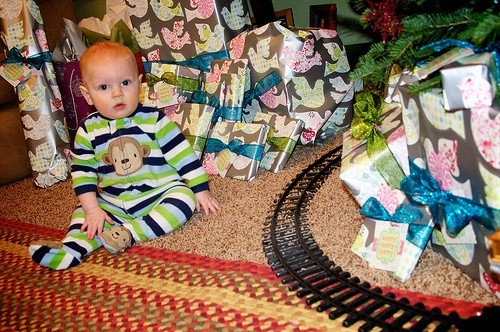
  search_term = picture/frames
[251,7,294,27]
[309,3,337,29]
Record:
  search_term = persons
[28,40,221,271]
